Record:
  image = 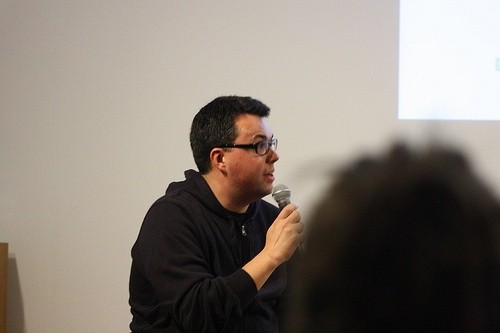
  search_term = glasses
[217,138,278,155]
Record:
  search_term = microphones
[272,185,304,250]
